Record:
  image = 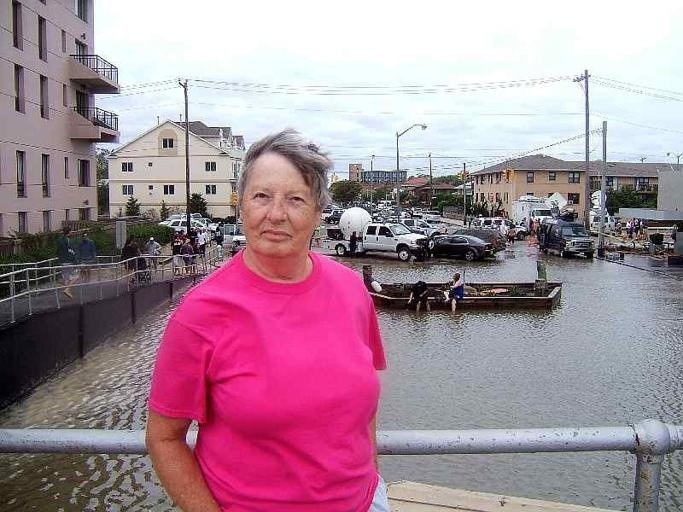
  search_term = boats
[362,260,562,308]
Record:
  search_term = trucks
[512,195,558,232]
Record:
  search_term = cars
[324,201,448,235]
[434,235,495,261]
[158,213,220,241]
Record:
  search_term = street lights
[396,123,427,203]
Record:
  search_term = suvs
[453,226,506,253]
[224,223,246,256]
[471,216,527,241]
[538,221,595,258]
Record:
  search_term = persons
[508,226,515,246]
[614,217,648,240]
[78,233,97,282]
[57,227,80,298]
[234,241,242,256]
[443,273,464,312]
[527,214,542,245]
[314,228,320,245]
[146,129,390,512]
[349,231,358,258]
[119,223,223,286]
[408,281,430,312]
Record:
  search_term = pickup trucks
[323,222,428,261]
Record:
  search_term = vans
[590,216,611,236]
